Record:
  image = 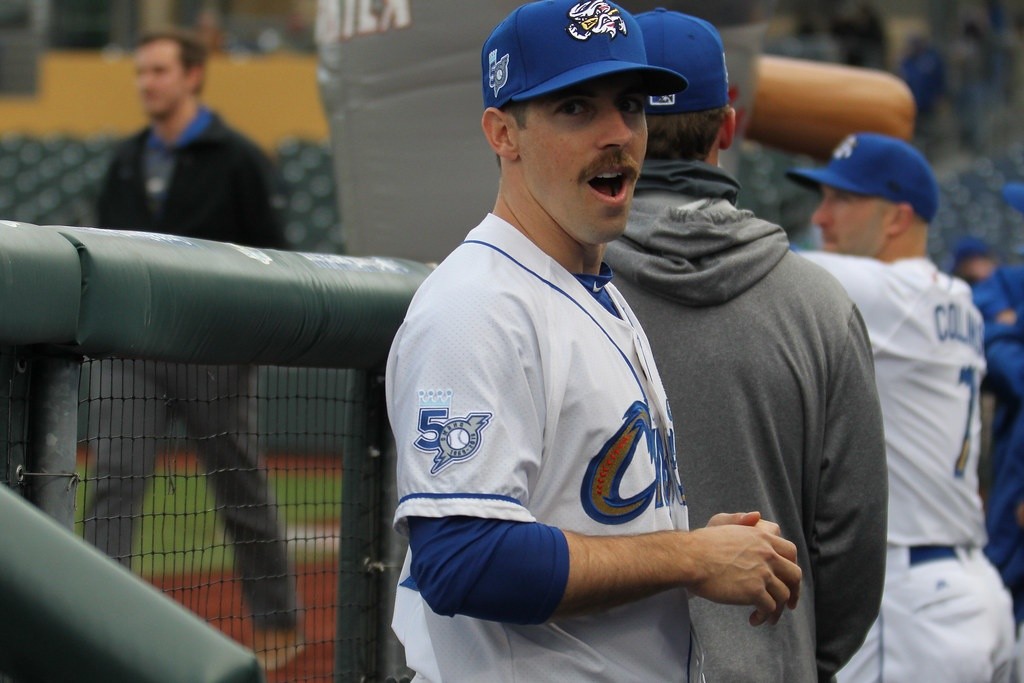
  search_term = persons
[79,33,309,671]
[602,6,890,683]
[385,1,803,683]
[789,133,1024,683]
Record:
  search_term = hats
[946,237,987,276]
[632,8,730,114]
[787,133,938,223]
[481,1,689,111]
[1002,180,1024,215]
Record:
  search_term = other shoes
[250,637,305,670]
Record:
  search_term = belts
[910,545,956,567]
